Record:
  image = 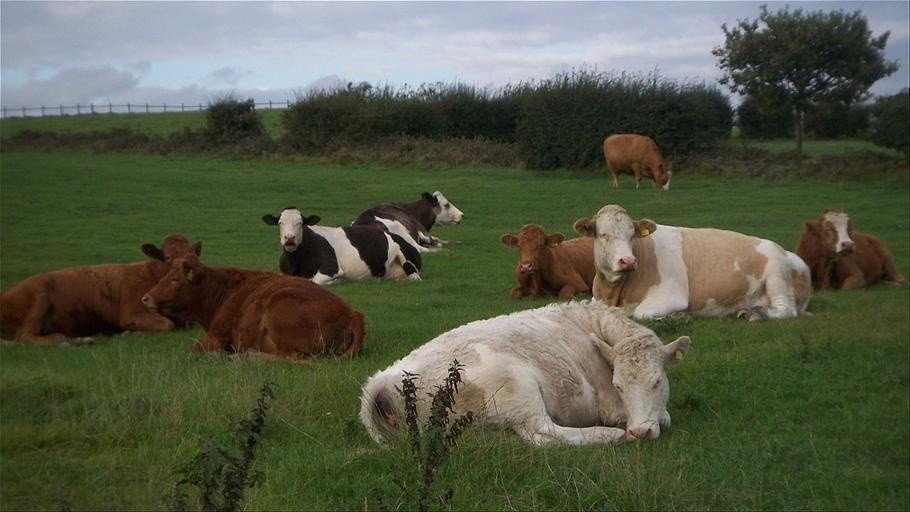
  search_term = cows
[796,208,904,291]
[261,190,469,288]
[141,254,366,366]
[358,294,692,453]
[2,234,202,348]
[501,225,596,300]
[602,132,674,191]
[574,205,813,324]
